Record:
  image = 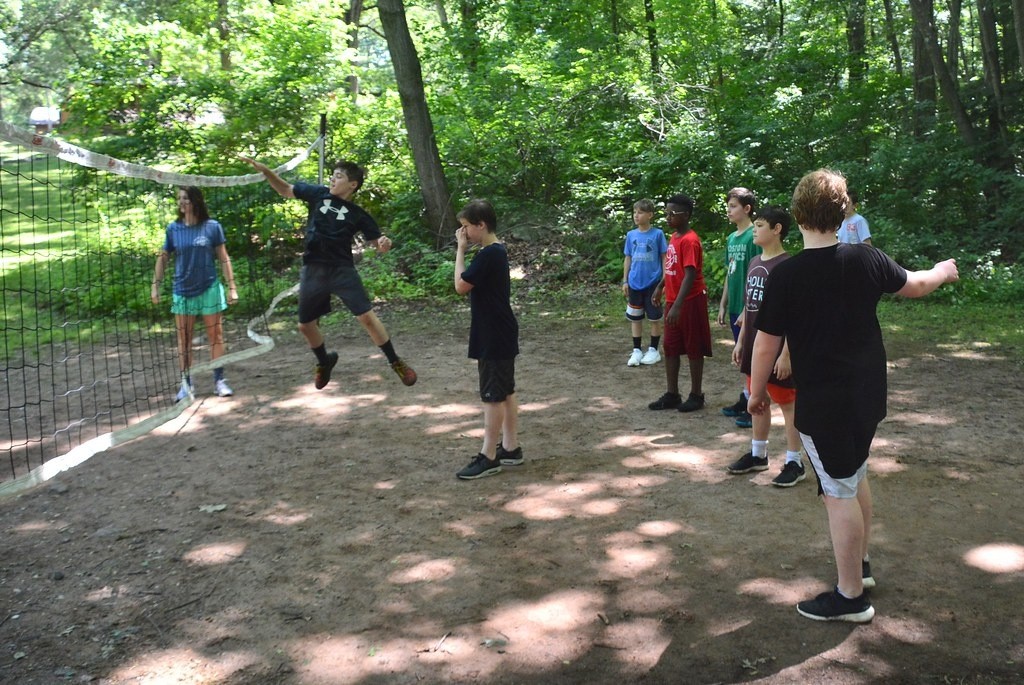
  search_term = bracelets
[622,281,627,284]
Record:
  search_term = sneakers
[795,586,875,622]
[862,556,876,588]
[640,347,662,365]
[723,393,749,415]
[678,392,705,412]
[496,443,524,465]
[213,378,234,396]
[174,385,194,404]
[727,451,769,474]
[735,411,752,427]
[647,392,682,410]
[456,453,501,479]
[771,460,806,487]
[627,348,643,366]
[315,352,339,389]
[392,358,418,386]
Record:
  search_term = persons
[648,195,709,413]
[621,198,667,366]
[714,171,958,624]
[236,152,417,391]
[454,202,523,480]
[149,184,237,401]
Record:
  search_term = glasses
[664,211,685,217]
